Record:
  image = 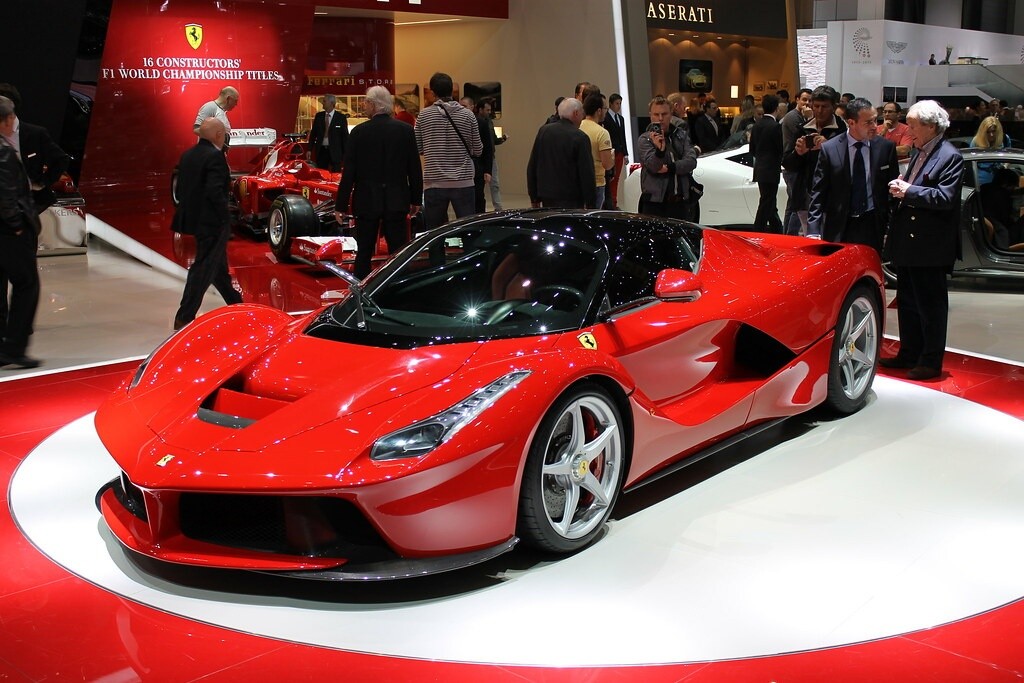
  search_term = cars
[895,130,1024,280]
[615,142,787,230]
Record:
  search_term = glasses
[600,106,609,111]
[709,106,719,111]
[882,110,897,115]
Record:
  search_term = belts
[848,209,877,218]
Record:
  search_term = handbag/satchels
[469,155,488,178]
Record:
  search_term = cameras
[652,122,662,134]
[805,135,815,148]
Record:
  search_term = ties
[850,141,868,217]
[324,113,331,138]
[614,114,619,128]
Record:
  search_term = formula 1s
[168,131,423,272]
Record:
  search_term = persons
[193,86,239,157]
[637,96,704,224]
[970,116,1011,187]
[724,85,913,254]
[476,99,509,210]
[0,82,59,368]
[414,73,483,267]
[334,86,424,280]
[526,82,629,210]
[306,94,349,173]
[393,98,414,126]
[668,92,724,155]
[879,100,964,380]
[947,99,1024,122]
[460,97,493,214]
[170,117,243,330]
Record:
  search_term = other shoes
[908,366,942,379]
[0,351,39,368]
[878,356,916,369]
[174,319,193,330]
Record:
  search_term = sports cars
[93,206,887,583]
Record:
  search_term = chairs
[492,248,557,300]
